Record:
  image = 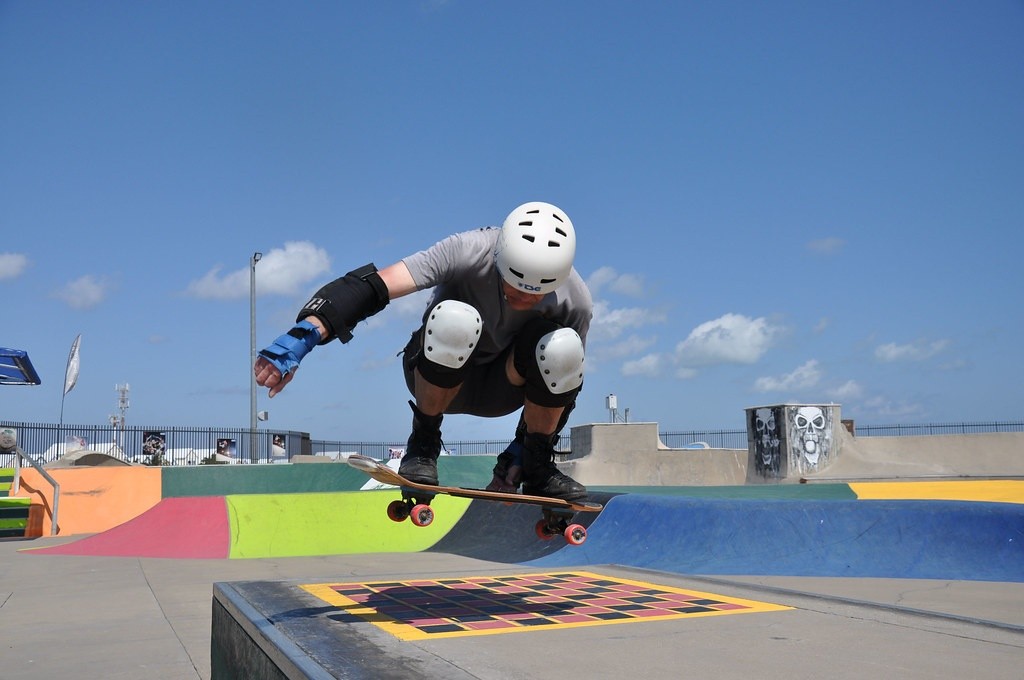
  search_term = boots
[398,400,446,487]
[493,429,588,501]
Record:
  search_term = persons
[254,202,593,500]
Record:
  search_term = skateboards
[346,454,603,546]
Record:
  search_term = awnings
[0,347,41,385]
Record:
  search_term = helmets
[493,202,576,295]
